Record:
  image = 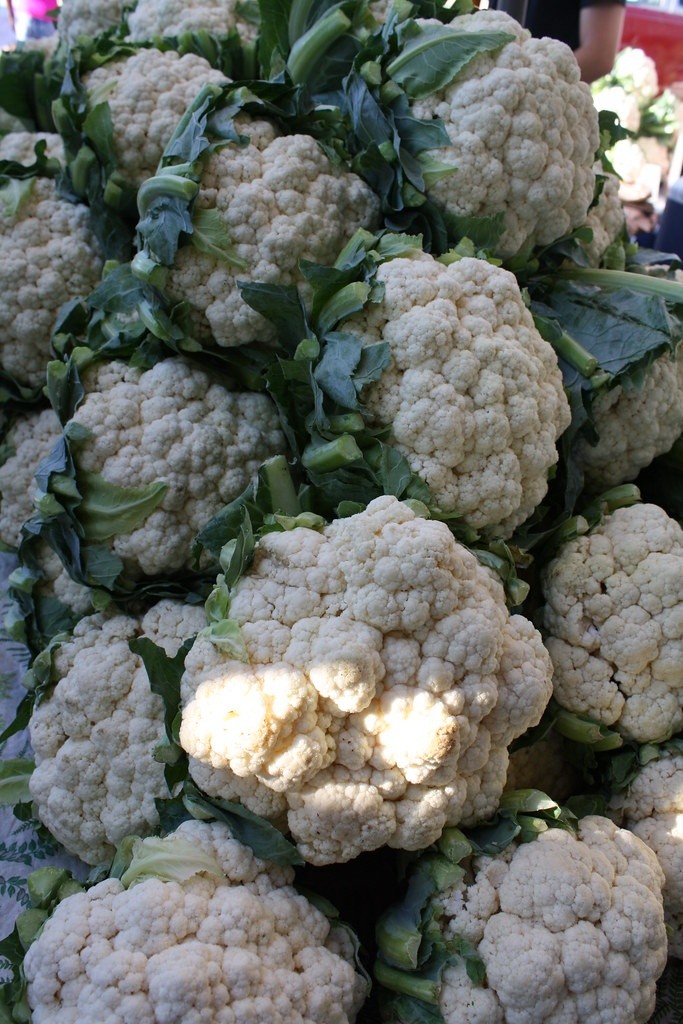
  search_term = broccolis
[0,0,683,1024]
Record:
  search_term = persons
[489,0,628,84]
